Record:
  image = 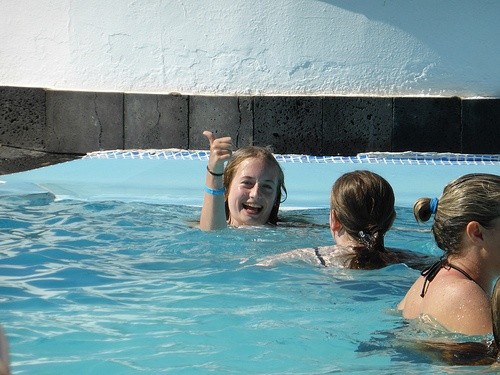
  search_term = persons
[243,171,441,272]
[199,130,287,232]
[419,277,500,365]
[396,173,500,337]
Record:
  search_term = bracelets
[205,184,228,196]
[206,166,225,178]
